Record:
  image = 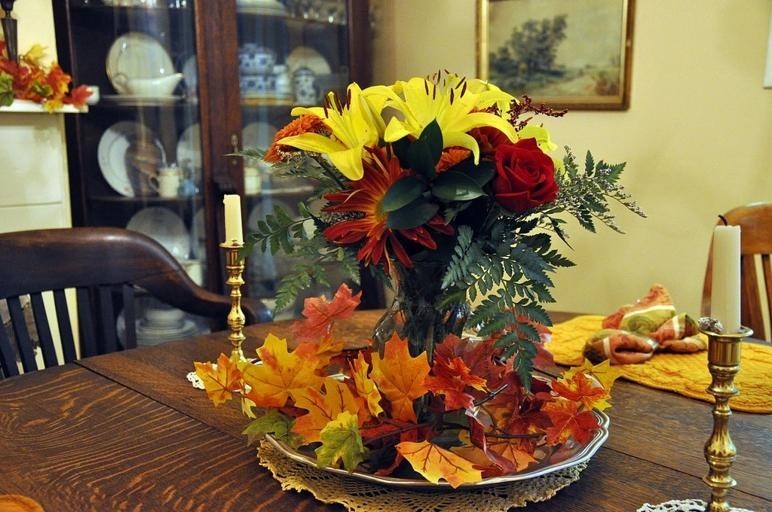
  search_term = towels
[543,313,772,415]
[582,284,708,364]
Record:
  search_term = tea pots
[113,71,182,96]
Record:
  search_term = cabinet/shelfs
[52,0,387,359]
[0,0,88,376]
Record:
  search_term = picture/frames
[474,0,636,111]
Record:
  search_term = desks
[0,311,772,512]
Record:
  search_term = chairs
[0,227,259,385]
[701,201,772,348]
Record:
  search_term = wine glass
[283,0,348,25]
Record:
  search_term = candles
[711,215,741,336]
[224,193,243,247]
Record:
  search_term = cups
[182,258,204,285]
[83,86,100,106]
[292,69,324,105]
[148,167,184,198]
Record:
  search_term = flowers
[233,69,647,446]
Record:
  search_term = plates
[192,206,206,263]
[238,1,288,17]
[286,47,333,96]
[105,31,175,95]
[128,322,199,349]
[101,94,187,108]
[175,46,199,108]
[126,208,191,261]
[176,123,204,192]
[250,199,298,276]
[242,122,281,182]
[113,298,213,347]
[98,120,168,199]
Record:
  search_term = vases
[371,297,470,449]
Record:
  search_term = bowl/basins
[237,44,286,94]
[145,306,188,324]
